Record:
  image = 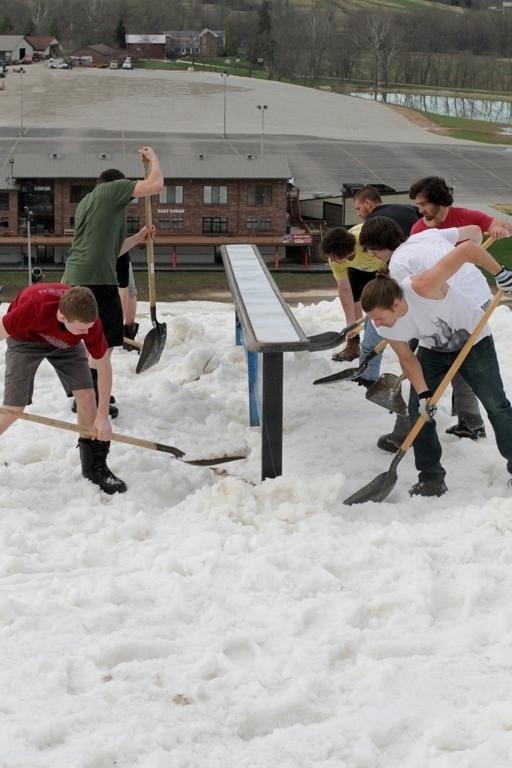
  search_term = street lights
[220,70,230,137]
[255,103,269,155]
[16,67,28,139]
[23,203,36,288]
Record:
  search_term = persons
[59,143,164,419]
[1,281,126,494]
[361,216,488,453]
[410,175,511,237]
[353,185,423,391]
[360,238,512,495]
[116,254,138,351]
[320,221,384,361]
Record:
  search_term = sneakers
[407,482,448,496]
[377,432,413,453]
[444,424,488,440]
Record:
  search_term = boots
[72,369,119,418]
[331,333,361,361]
[76,438,127,494]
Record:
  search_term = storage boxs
[293,234,312,243]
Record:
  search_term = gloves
[417,391,438,424]
[494,267,512,294]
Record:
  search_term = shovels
[342,290,505,506]
[366,372,411,418]
[313,236,495,385]
[136,149,166,374]
[0,408,251,466]
[307,316,367,351]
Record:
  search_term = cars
[0,54,137,72]
